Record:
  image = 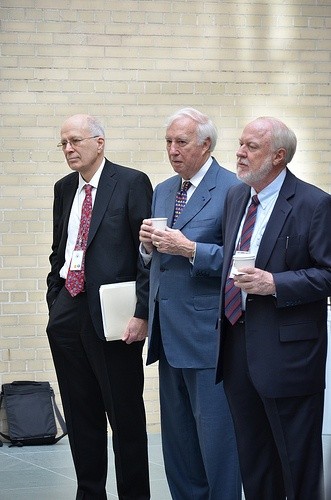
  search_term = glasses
[57,136,99,150]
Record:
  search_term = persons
[139,106,243,500]
[213,116,331,499]
[46,112,153,500]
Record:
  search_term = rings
[155,242,159,248]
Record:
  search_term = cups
[149,218,168,245]
[233,255,256,274]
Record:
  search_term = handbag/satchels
[0,380,58,445]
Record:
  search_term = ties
[172,181,192,226]
[64,184,94,298]
[225,195,260,326]
